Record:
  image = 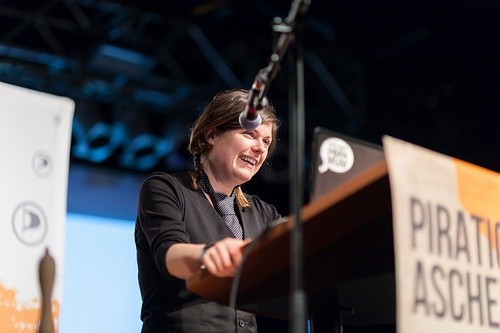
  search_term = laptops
[308,126,384,202]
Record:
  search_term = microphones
[239,69,262,131]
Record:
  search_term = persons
[135,89,282,333]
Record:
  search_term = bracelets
[199,239,218,271]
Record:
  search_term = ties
[196,168,243,242]
